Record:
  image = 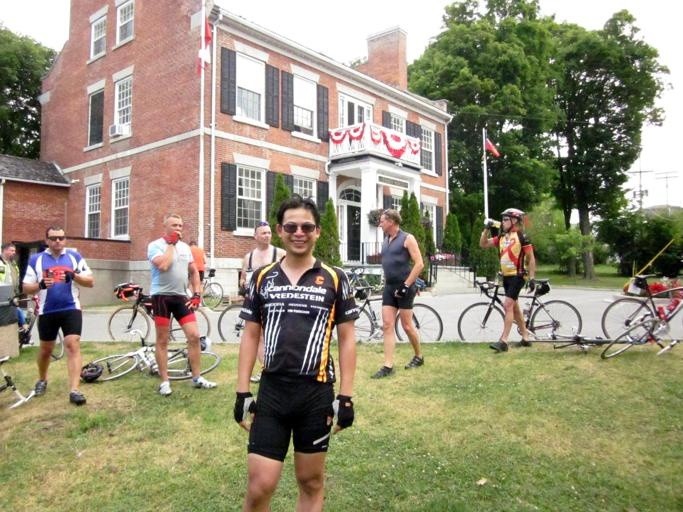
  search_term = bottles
[483,219,501,228]
[44,268,53,289]
[26,307,34,324]
[666,299,679,312]
[656,307,666,321]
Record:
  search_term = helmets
[501,207,524,220]
[79,361,103,382]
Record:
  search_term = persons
[145,212,217,396]
[20,224,94,407]
[477,207,536,352]
[10,298,33,345]
[186,239,206,308]
[238,220,287,383]
[231,192,359,511]
[368,208,425,381]
[0,242,19,306]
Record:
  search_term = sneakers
[371,364,395,379]
[70,391,86,405]
[405,354,424,369]
[33,379,49,396]
[515,339,532,347]
[157,381,172,395]
[192,376,216,389]
[490,340,509,352]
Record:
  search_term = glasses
[282,222,316,234]
[48,236,66,242]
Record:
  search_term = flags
[482,131,501,158]
[193,15,211,78]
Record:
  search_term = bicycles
[0,354,37,413]
[79,325,222,384]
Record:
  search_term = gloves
[233,391,257,424]
[38,279,48,290]
[329,394,356,430]
[190,293,200,306]
[526,279,535,291]
[397,282,410,297]
[167,232,180,243]
[63,270,75,284]
